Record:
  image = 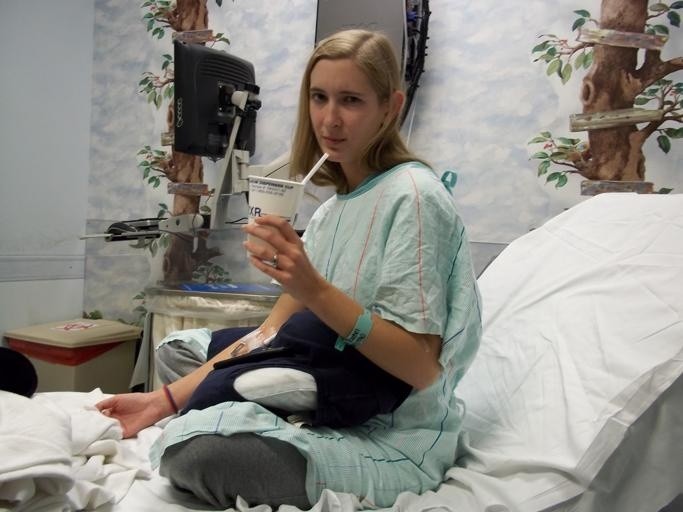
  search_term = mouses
[105,221,134,236]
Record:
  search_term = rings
[272,254,279,268]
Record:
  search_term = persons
[92,28,483,509]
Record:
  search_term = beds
[1,190,682,509]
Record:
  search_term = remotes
[213,346,290,370]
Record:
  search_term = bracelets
[162,384,179,413]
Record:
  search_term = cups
[248,174,304,259]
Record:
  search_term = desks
[130,288,281,389]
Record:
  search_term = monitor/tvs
[171,38,260,156]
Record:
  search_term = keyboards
[131,218,162,226]
